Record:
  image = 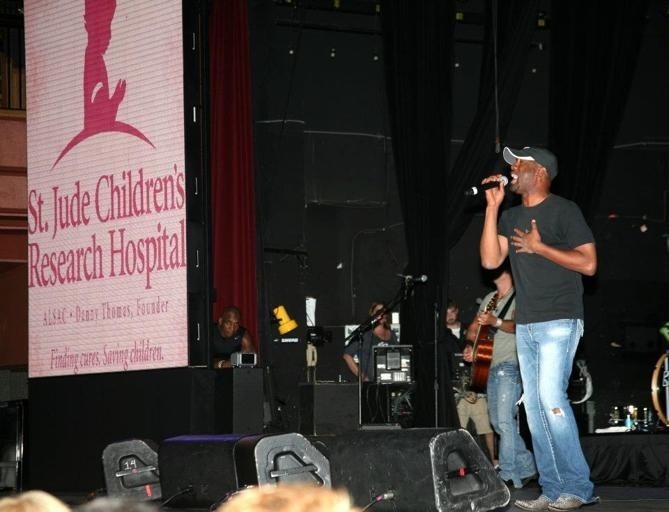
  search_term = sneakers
[547,495,582,511]
[514,495,550,512]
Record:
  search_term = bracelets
[495,317,503,329]
[217,360,226,369]
[465,339,475,349]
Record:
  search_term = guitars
[466,294,494,390]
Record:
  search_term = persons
[208,479,364,512]
[450,331,502,466]
[75,494,171,512]
[475,267,539,490]
[436,297,468,373]
[0,488,73,512]
[342,301,410,427]
[460,141,602,512]
[212,305,255,370]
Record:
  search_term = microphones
[463,176,509,198]
[408,275,428,284]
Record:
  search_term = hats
[503,146,558,180]
